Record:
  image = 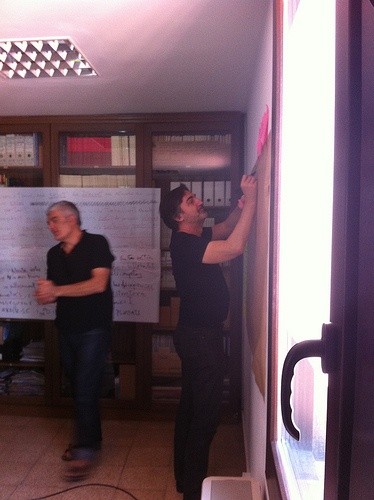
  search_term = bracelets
[238,200,245,210]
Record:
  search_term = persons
[32,200,117,481]
[160,175,259,500]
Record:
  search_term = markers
[249,168,257,181]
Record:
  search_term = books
[0,134,230,170]
[153,217,233,408]
[59,175,232,209]
[0,324,135,401]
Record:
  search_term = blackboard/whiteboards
[0,188,160,323]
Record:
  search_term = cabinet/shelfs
[0,111,246,423]
